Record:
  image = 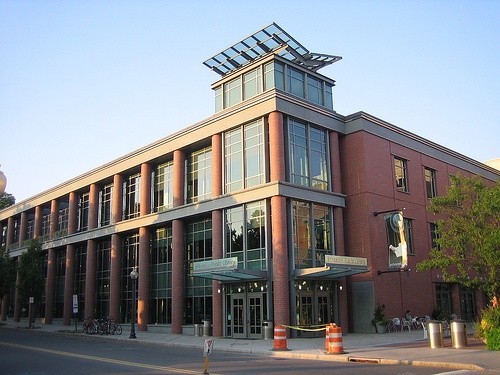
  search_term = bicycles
[83,315,123,336]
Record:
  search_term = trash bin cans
[450,319,467,348]
[194,324,200,337]
[427,320,444,348]
[261,320,273,340]
[202,319,211,337]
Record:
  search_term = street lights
[129,270,139,338]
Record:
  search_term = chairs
[384,314,457,340]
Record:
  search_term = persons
[405,309,421,329]
